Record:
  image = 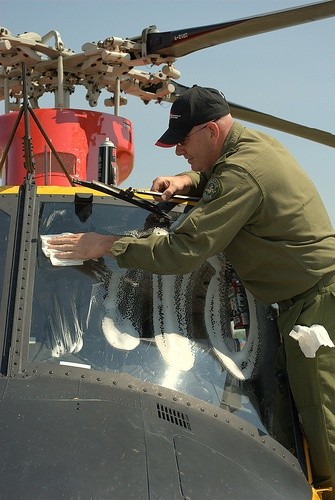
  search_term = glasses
[176,117,221,148]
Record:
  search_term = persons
[47,83,335,500]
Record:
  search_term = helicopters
[0,0,335,500]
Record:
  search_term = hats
[154,84,232,148]
